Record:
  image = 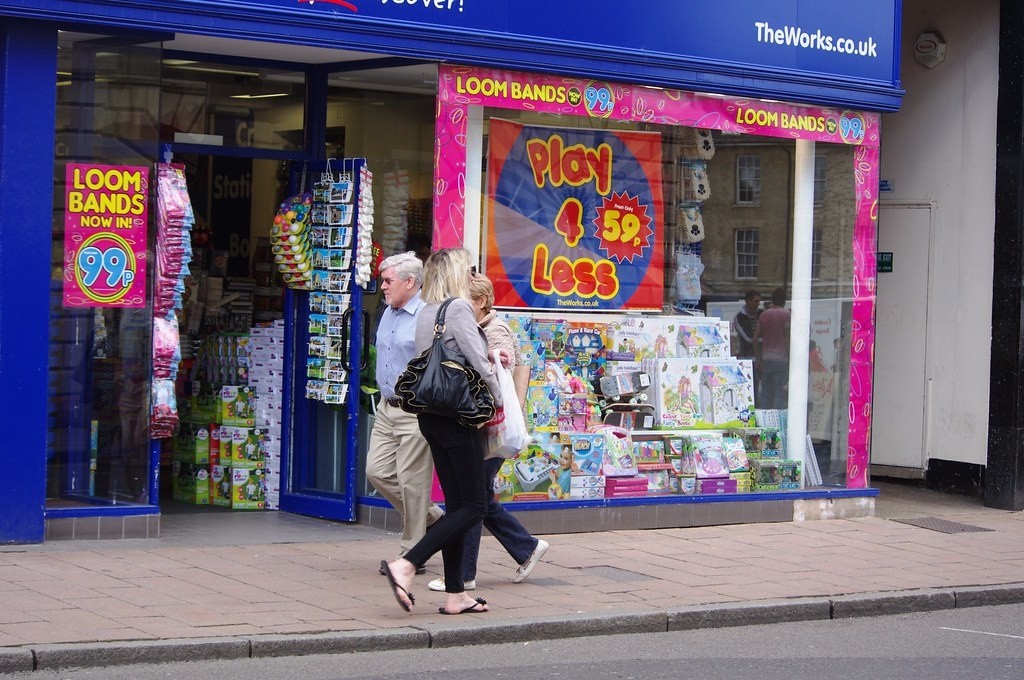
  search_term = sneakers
[512,539,549,583]
[428,576,476,591]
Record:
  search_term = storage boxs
[173,320,284,511]
[493,314,802,502]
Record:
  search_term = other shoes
[378,562,426,575]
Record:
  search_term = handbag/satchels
[482,348,534,460]
[394,297,496,428]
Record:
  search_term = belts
[386,397,402,407]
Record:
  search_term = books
[306,182,353,401]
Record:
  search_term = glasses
[469,266,477,276]
[379,277,418,285]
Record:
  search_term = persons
[256,475,264,498]
[191,429,197,450]
[211,389,218,409]
[380,246,512,615]
[366,249,444,574]
[180,287,194,306]
[429,272,548,591]
[192,470,197,485]
[235,396,247,418]
[245,439,256,460]
[255,435,265,458]
[222,477,230,499]
[245,392,254,415]
[246,479,258,501]
[734,287,838,412]
[550,444,572,494]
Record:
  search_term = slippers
[381,560,415,612]
[439,597,488,615]
